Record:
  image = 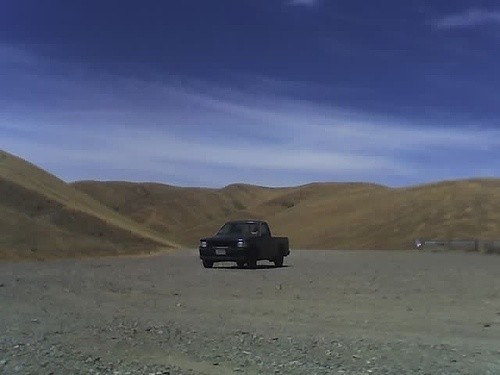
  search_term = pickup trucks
[198,220,290,268]
